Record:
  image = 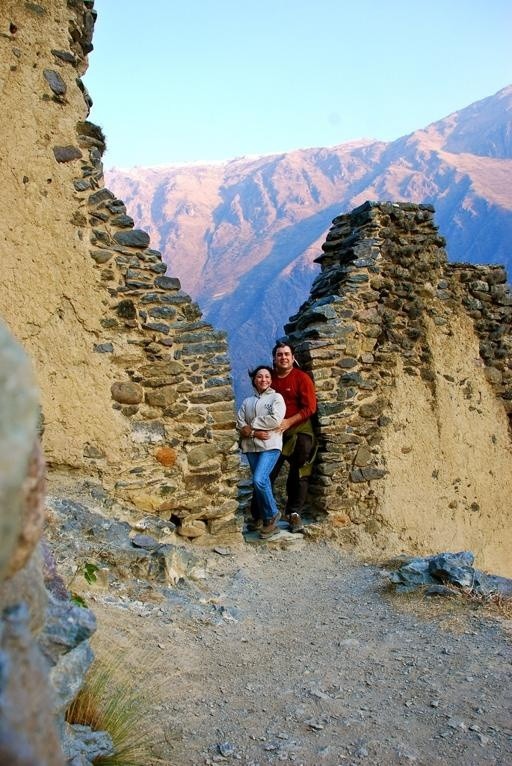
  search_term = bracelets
[248,429,255,438]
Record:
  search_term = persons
[234,364,288,533]
[259,341,318,541]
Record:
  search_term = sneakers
[247,512,282,539]
[288,514,302,532]
